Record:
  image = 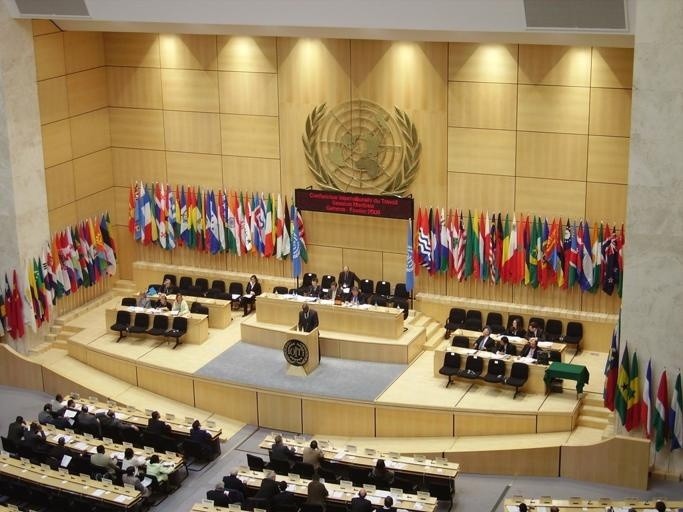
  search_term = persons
[137,279,191,314]
[298,304,321,363]
[241,275,261,315]
[307,265,364,304]
[217,435,396,511]
[473,316,542,360]
[656,502,665,511]
[519,503,559,512]
[8,394,215,494]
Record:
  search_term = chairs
[439,308,583,398]
[110,274,263,348]
[272,272,409,333]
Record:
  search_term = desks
[501,491,682,512]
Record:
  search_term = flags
[129,181,308,278]
[0,210,130,356]
[603,325,683,452]
[406,205,624,297]
